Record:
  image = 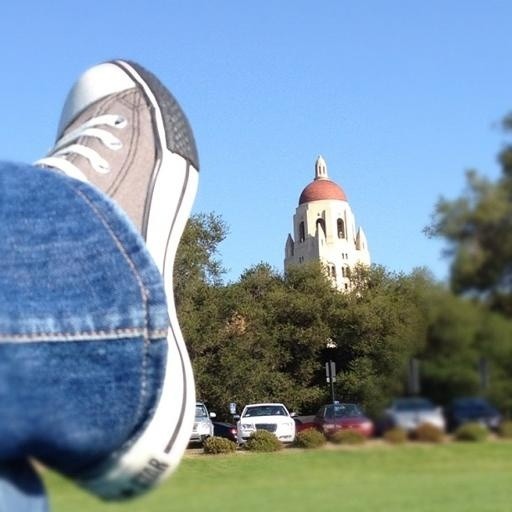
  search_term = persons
[0,61,199,512]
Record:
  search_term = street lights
[326,335,343,435]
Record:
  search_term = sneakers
[32,58,203,505]
